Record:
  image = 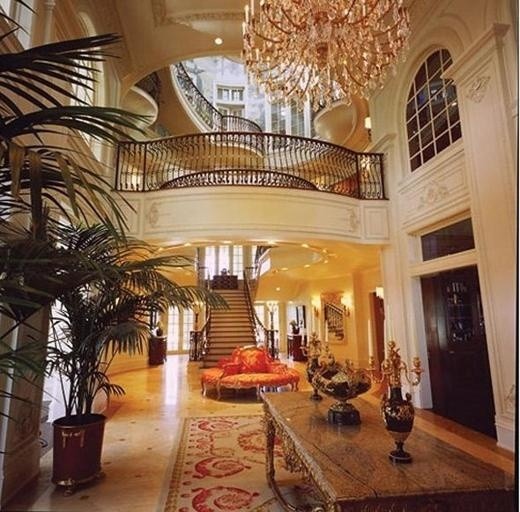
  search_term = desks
[259,387,516,512]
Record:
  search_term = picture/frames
[150,301,156,329]
[295,305,306,328]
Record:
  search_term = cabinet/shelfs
[148,336,167,364]
[213,275,238,290]
[286,333,308,361]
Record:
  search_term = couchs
[200,345,301,402]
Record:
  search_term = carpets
[163,414,324,512]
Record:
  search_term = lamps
[240,0,410,113]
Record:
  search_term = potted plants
[16,209,231,496]
